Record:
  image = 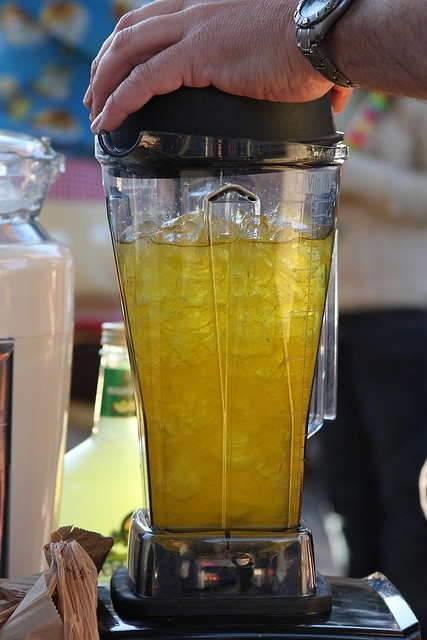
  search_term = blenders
[97,89,418,639]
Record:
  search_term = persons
[83,1,427,136]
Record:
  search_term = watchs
[293,0,355,94]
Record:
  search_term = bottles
[53,322,152,585]
[0,130,75,582]
